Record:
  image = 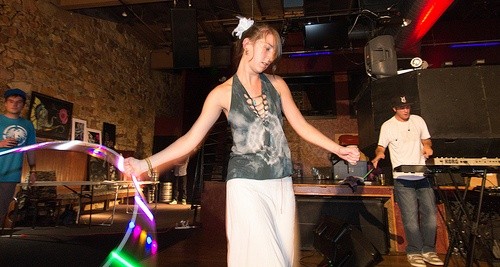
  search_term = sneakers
[406,252,426,267]
[421,248,444,265]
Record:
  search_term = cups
[377,174,385,185]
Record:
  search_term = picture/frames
[87,155,117,190]
[28,91,74,140]
[71,118,116,149]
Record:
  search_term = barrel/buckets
[160,183,173,202]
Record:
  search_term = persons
[0,89,37,228]
[124,18,360,267]
[371,96,444,267]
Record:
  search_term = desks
[293,176,398,255]
[2,180,162,238]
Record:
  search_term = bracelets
[144,157,152,171]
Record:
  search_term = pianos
[426,156,500,267]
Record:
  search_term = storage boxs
[332,160,369,182]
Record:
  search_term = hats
[5,89,26,103]
[392,95,414,106]
